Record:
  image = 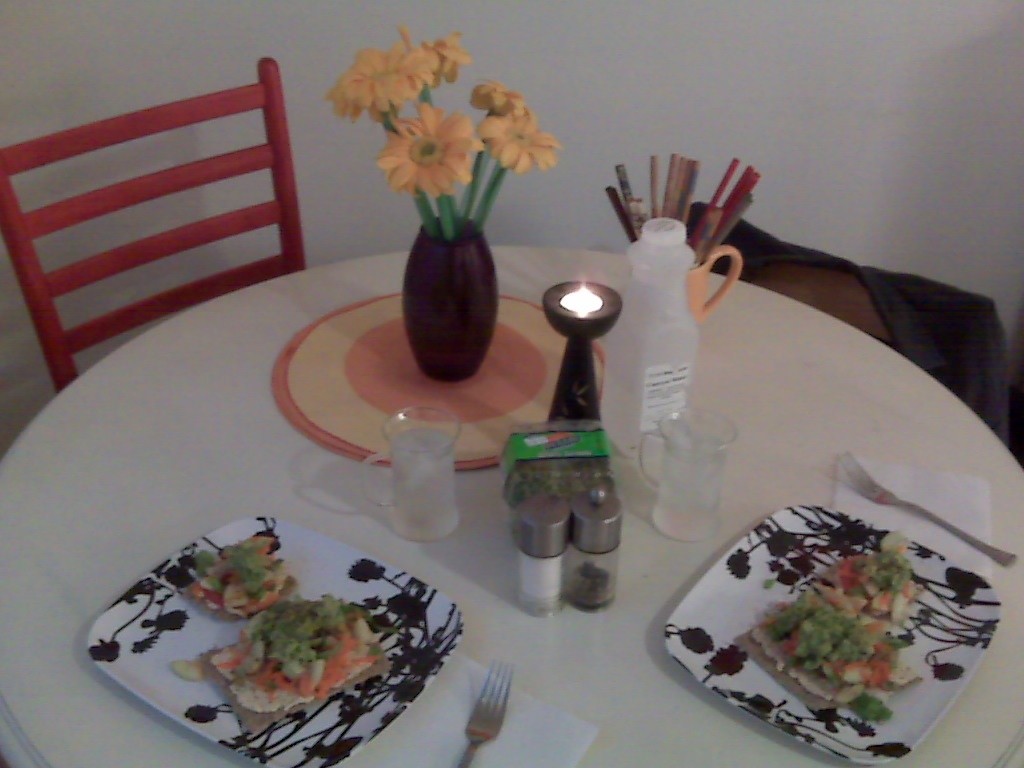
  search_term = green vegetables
[193,540,401,694]
[760,549,917,722]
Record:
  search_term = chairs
[686,201,1010,445]
[0,53,306,398]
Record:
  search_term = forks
[836,449,1018,569]
[457,667,514,768]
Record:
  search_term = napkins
[821,449,994,580]
[339,653,600,768]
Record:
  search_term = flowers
[325,25,564,234]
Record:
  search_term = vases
[400,216,501,386]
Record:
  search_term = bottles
[599,217,697,453]
[569,487,625,610]
[516,496,569,614]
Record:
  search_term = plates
[664,502,1000,768]
[88,516,465,768]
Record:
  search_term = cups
[361,404,465,540]
[688,244,743,328]
[637,409,743,539]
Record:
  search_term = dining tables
[0,238,1024,768]
